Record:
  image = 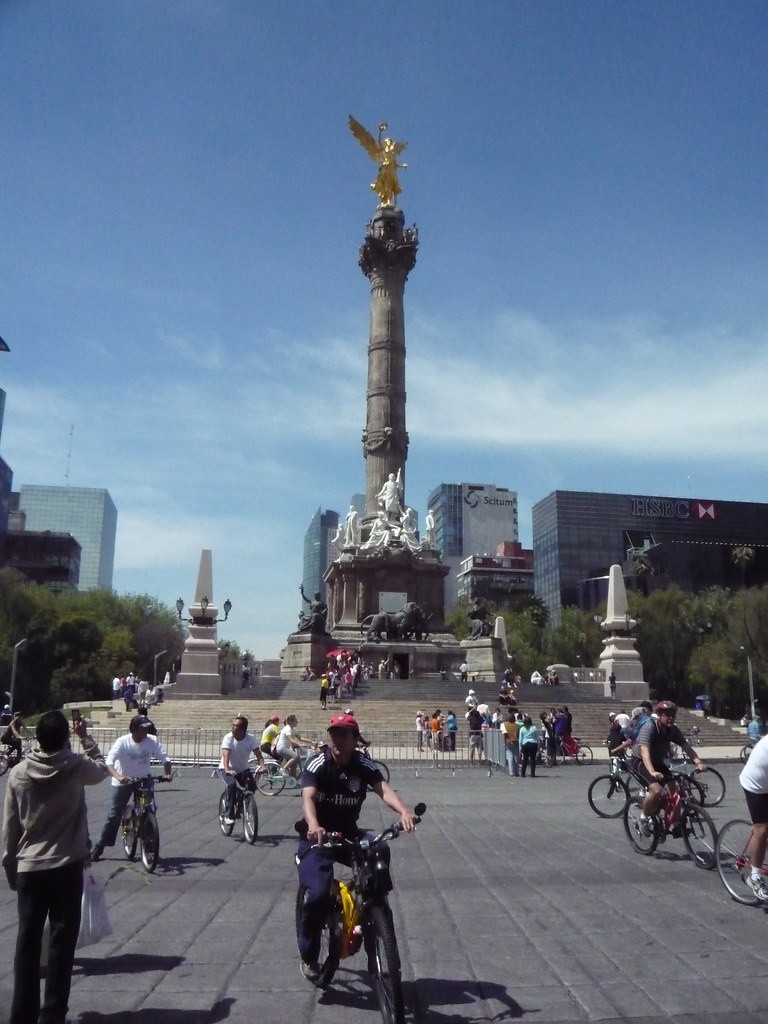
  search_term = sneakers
[746,874,768,901]
[635,814,651,837]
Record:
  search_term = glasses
[230,723,242,727]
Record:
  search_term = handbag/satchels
[74,864,113,950]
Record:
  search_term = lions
[360,602,433,642]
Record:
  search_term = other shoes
[298,949,320,982]
[280,767,290,779]
[223,816,235,826]
[4,753,9,760]
[91,845,103,861]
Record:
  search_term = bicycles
[536,734,593,766]
[739,743,761,763]
[1,734,40,773]
[293,801,427,1024]
[219,770,261,845]
[718,817,768,905]
[255,748,325,797]
[624,766,718,870]
[119,775,174,872]
[352,738,389,789]
[588,748,728,819]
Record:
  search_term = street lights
[8,638,28,729]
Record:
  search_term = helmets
[656,701,678,711]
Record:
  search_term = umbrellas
[326,648,355,659]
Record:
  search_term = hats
[468,689,475,696]
[270,715,280,721]
[326,714,358,735]
[131,714,152,727]
[640,700,651,708]
[321,674,327,677]
[631,707,644,722]
[417,711,423,716]
[754,714,760,719]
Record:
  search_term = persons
[173,654,181,682]
[2,711,37,762]
[738,733,768,899]
[242,663,251,688]
[0,704,13,726]
[298,657,416,711]
[416,665,762,797]
[631,701,707,839]
[89,715,172,863]
[136,707,157,736]
[469,596,496,638]
[417,612,433,642]
[332,467,438,553]
[295,582,327,635]
[0,711,110,1024]
[459,661,469,682]
[112,669,170,713]
[296,714,417,980]
[219,717,267,825]
[260,708,372,787]
[371,122,403,208]
[38,741,93,979]
